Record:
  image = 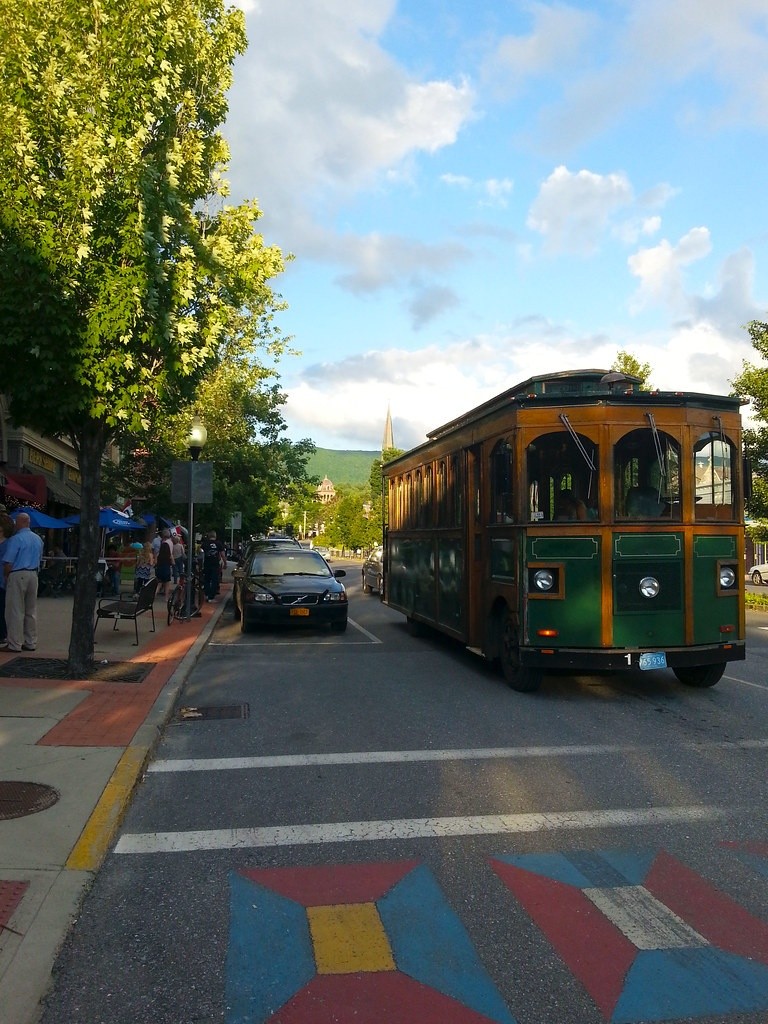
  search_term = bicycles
[167,570,205,626]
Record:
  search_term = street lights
[182,414,208,616]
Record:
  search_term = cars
[748,564,768,586]
[312,546,332,563]
[361,545,384,599]
[232,549,349,635]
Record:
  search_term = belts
[11,569,36,572]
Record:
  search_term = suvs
[237,537,304,568]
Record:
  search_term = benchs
[93,577,159,647]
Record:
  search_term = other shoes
[208,599,220,603]
[0,644,21,653]
[22,645,35,651]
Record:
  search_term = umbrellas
[11,507,73,528]
[61,505,174,528]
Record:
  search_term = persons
[152,522,228,602]
[104,544,122,595]
[200,531,227,604]
[133,542,153,599]
[47,546,67,597]
[0,514,17,647]
[559,489,588,521]
[0,512,44,653]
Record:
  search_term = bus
[380,370,753,696]
